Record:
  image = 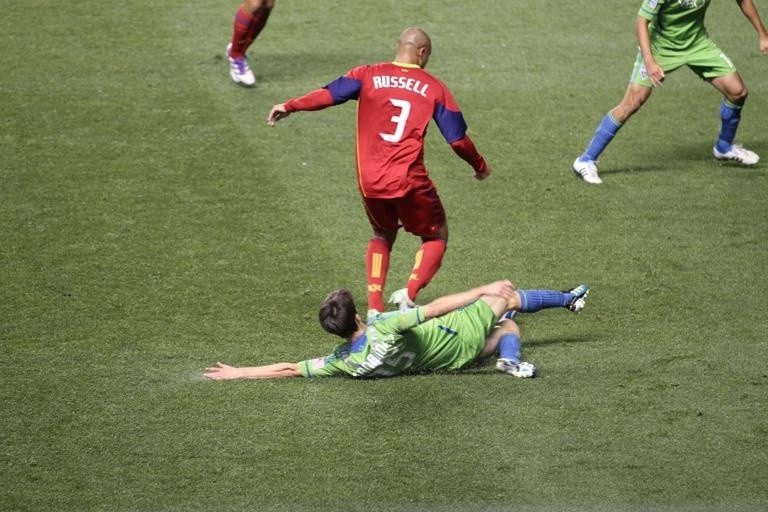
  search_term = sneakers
[388,287,416,312]
[573,157,603,185]
[366,308,381,326]
[714,143,760,165]
[565,284,589,314]
[495,357,536,379]
[225,42,256,86]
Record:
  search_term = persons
[204,278,590,379]
[266,28,492,327]
[223,0,278,86]
[573,0,767,184]
